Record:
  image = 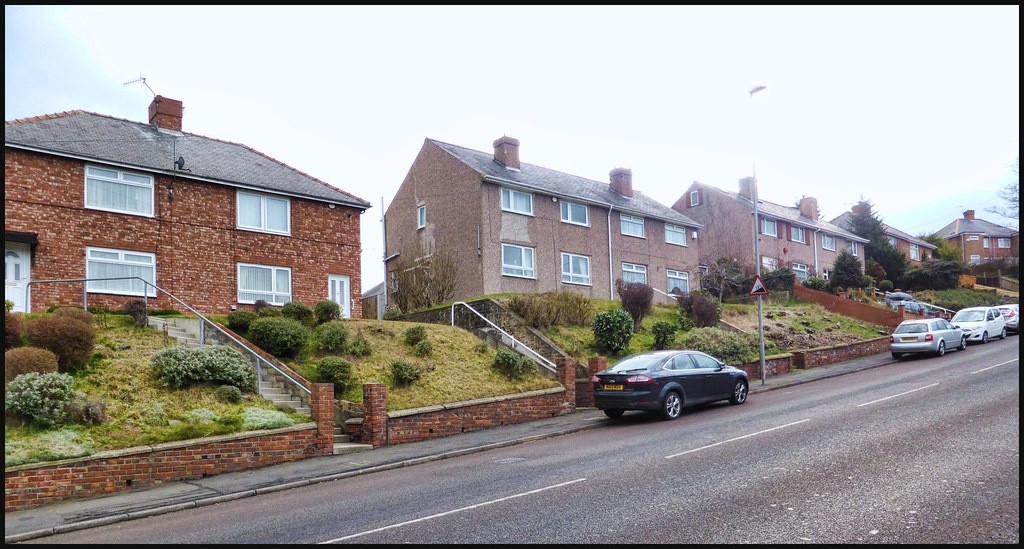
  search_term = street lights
[748,84,768,385]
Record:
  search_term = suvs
[882,300,935,318]
[994,303,1018,335]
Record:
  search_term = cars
[593,349,749,419]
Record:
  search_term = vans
[889,317,967,359]
[949,306,1007,344]
